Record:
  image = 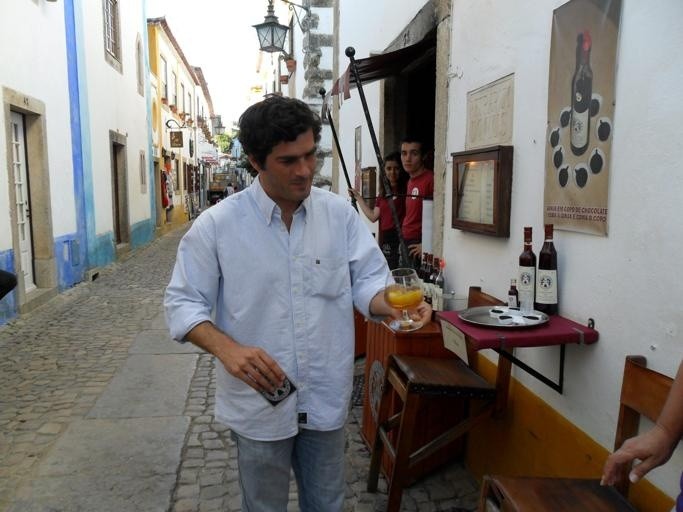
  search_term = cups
[519,287,536,316]
[547,92,611,192]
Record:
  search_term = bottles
[509,277,518,310]
[418,251,448,321]
[517,226,536,309]
[536,222,558,315]
[568,32,594,156]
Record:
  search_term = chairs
[479,355,675,512]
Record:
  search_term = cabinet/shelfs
[359,318,442,489]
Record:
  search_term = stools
[368,286,518,512]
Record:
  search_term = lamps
[251,0,308,53]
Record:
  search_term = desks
[436,305,599,395]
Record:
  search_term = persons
[345,149,409,272]
[222,182,234,196]
[161,94,433,512]
[396,135,434,284]
[598,359,683,512]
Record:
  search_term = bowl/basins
[490,310,540,326]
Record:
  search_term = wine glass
[385,268,425,333]
